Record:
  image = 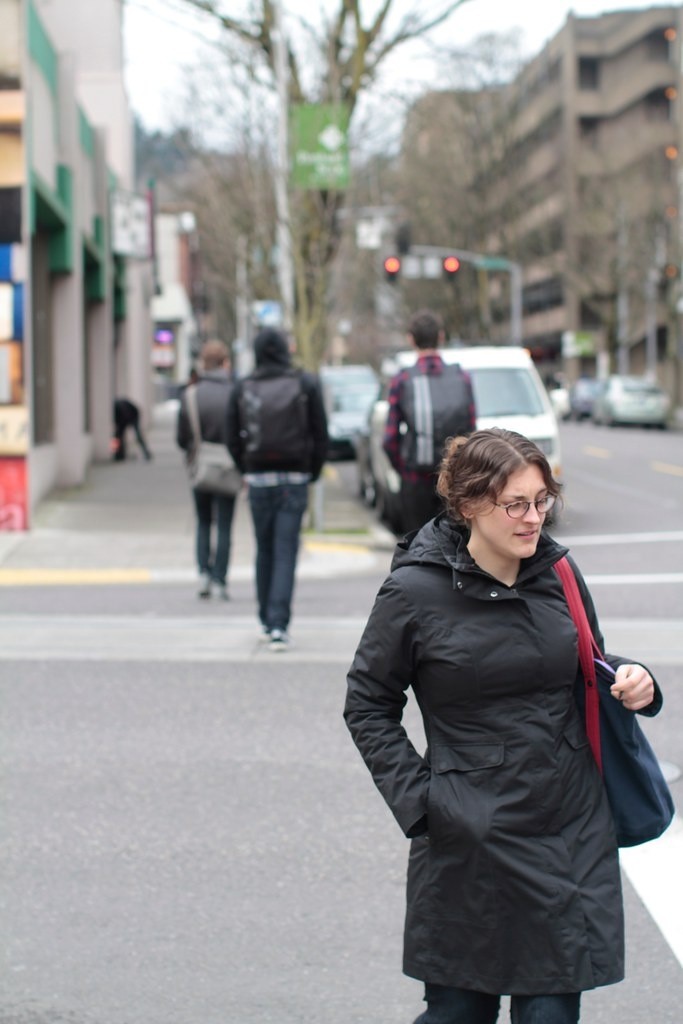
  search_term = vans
[363,343,564,525]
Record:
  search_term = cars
[575,377,594,423]
[315,364,382,385]
[544,374,572,420]
[593,375,674,424]
[322,384,384,458]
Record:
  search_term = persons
[226,331,328,649]
[342,427,664,1024]
[382,315,475,534]
[114,399,150,460]
[177,338,241,598]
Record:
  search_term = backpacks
[399,361,473,471]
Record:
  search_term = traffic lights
[384,258,401,284]
[445,256,460,279]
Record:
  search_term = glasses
[488,489,556,519]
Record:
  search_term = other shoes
[267,627,290,650]
[197,570,232,602]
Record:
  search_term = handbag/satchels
[554,554,675,846]
[191,441,242,496]
